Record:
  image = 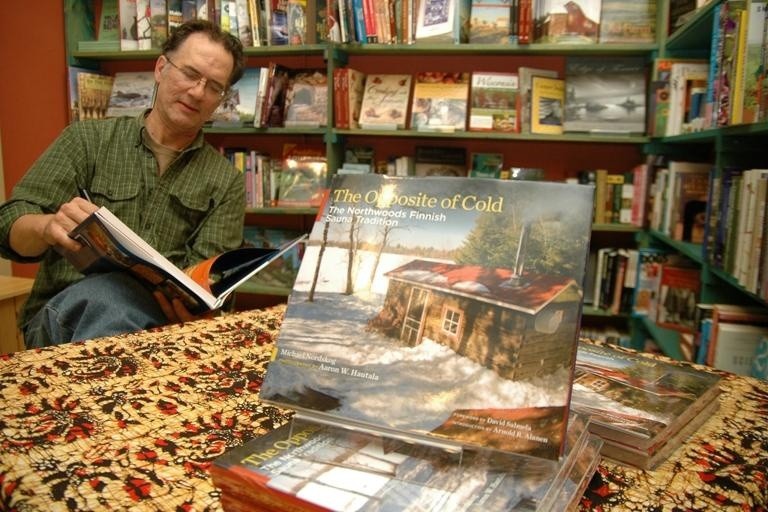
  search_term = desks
[0,275,36,354]
[0,303,767,512]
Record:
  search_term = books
[216,144,326,207]
[315,1,661,46]
[238,227,307,291]
[568,341,722,470]
[333,66,647,135]
[258,171,597,464]
[52,206,308,316]
[212,417,609,512]
[636,0,768,381]
[68,1,330,52]
[582,248,640,315]
[335,143,649,229]
[69,62,329,129]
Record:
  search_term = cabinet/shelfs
[63,2,768,371]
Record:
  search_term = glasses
[164,56,226,101]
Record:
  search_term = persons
[0,21,245,349]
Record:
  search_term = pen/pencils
[82,188,91,202]
